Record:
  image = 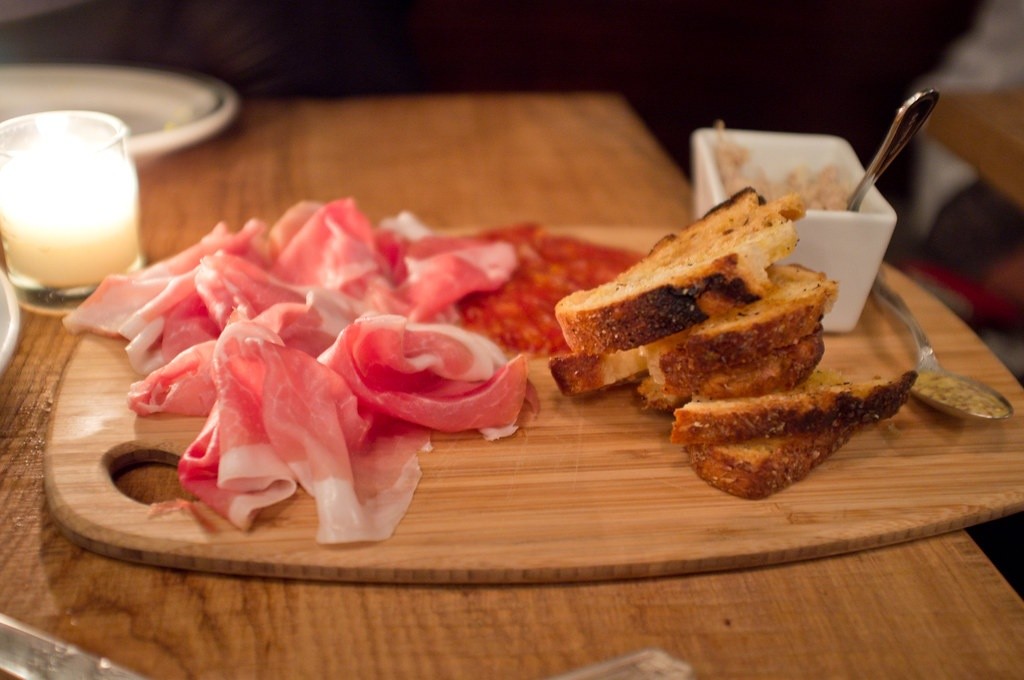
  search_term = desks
[4,91,1024,678]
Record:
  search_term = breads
[548,188,919,503]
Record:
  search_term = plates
[2,59,239,165]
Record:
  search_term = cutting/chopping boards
[43,222,1022,589]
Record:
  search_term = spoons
[870,273,1015,425]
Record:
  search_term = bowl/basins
[685,123,898,339]
